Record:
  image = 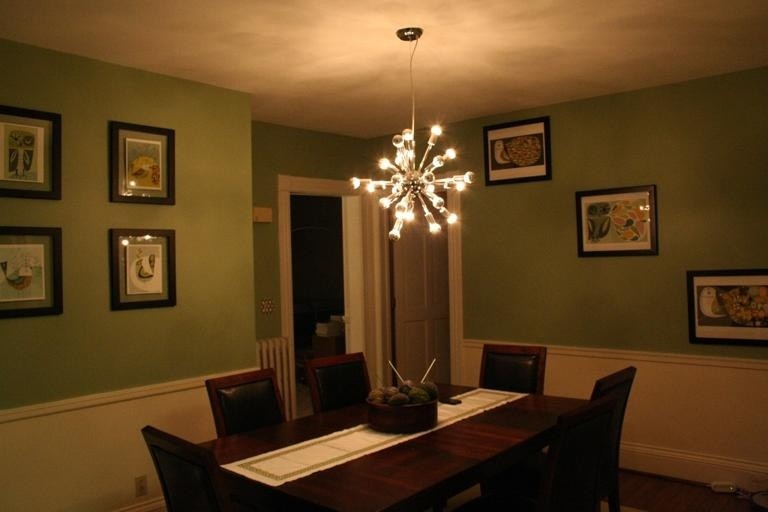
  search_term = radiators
[256,336,290,423]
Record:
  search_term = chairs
[204,367,287,439]
[479,393,614,512]
[140,424,222,510]
[588,366,638,512]
[479,343,547,394]
[304,352,372,414]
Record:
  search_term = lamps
[348,27,474,241]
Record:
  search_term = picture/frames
[108,120,175,205]
[686,269,768,346]
[574,184,658,258]
[109,228,176,311]
[0,226,63,319]
[0,104,62,200]
[482,116,552,187]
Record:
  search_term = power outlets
[135,475,148,497]
